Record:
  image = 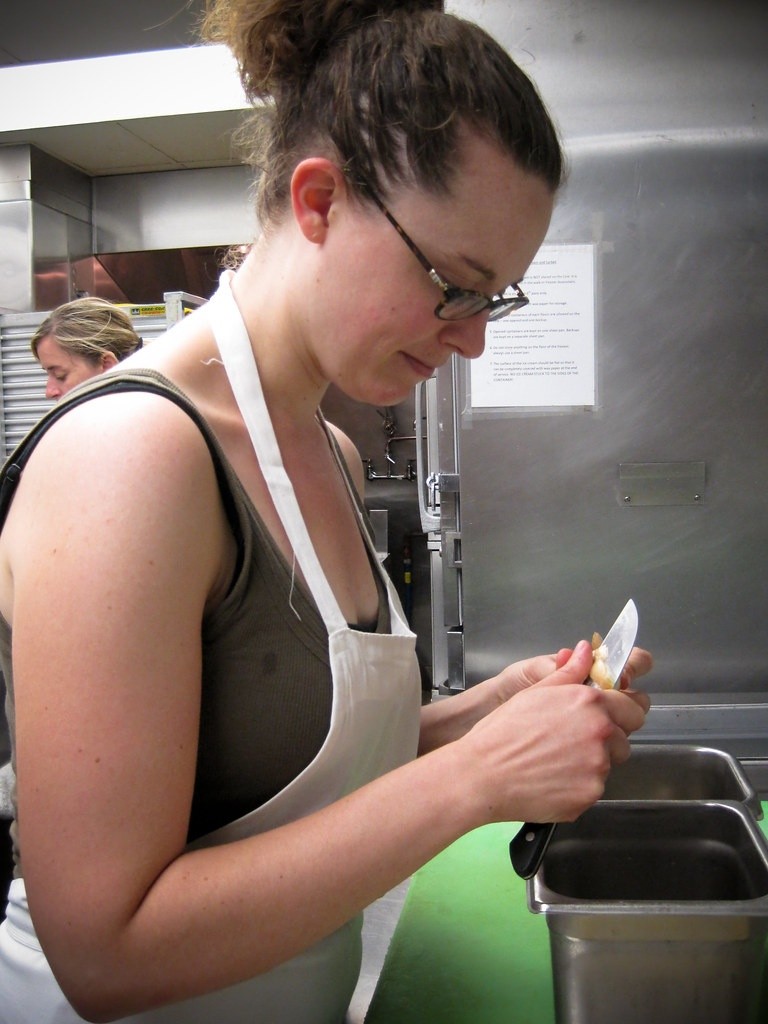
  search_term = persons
[31,296,142,403]
[2,2,652,1023]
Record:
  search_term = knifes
[510,599,638,879]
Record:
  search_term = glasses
[325,122,530,323]
